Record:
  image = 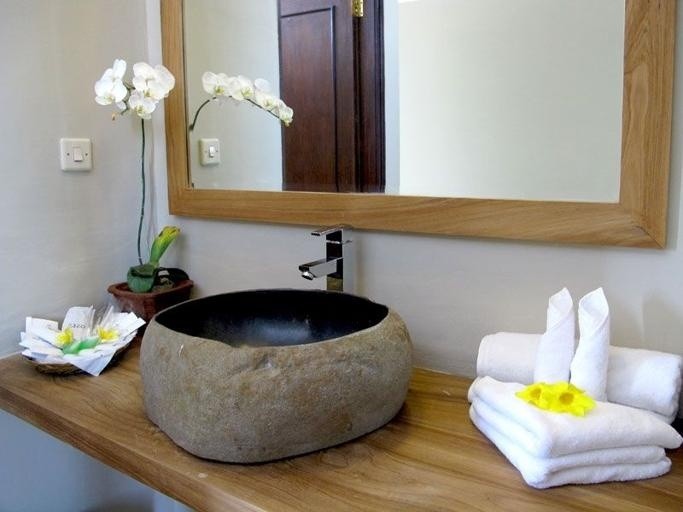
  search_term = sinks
[147,289,412,464]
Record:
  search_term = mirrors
[157,0,678,253]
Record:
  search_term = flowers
[90,51,189,295]
[183,70,297,135]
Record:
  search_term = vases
[108,276,197,324]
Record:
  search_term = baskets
[22,345,129,376]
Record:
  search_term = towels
[535,284,576,391]
[468,376,683,456]
[466,404,671,489]
[473,331,682,416]
[570,286,612,404]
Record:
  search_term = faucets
[297,223,353,291]
